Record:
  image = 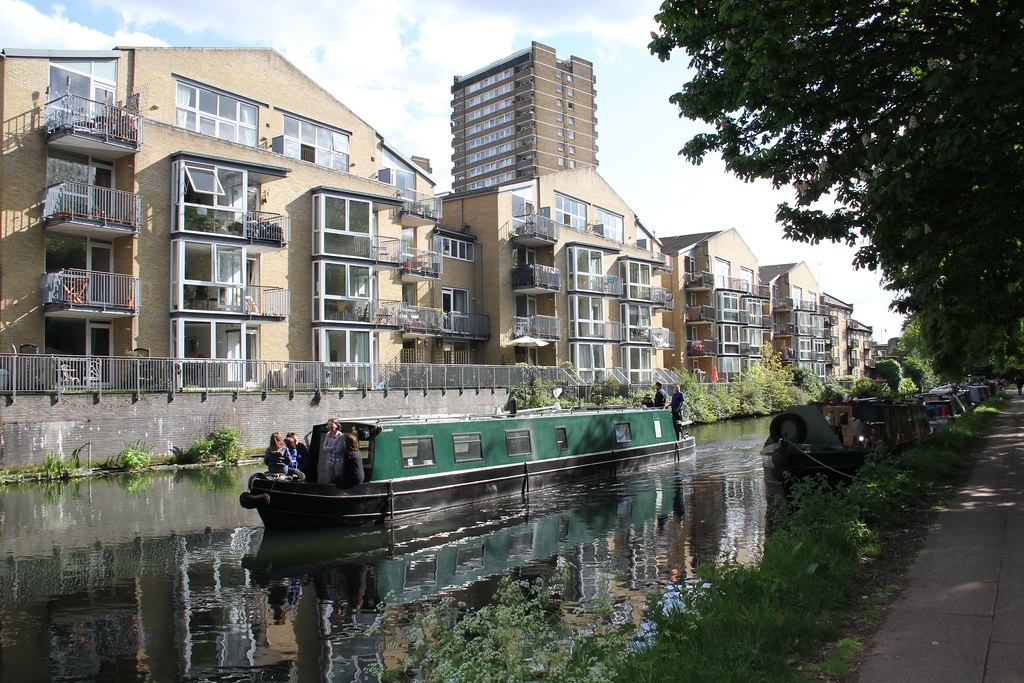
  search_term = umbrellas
[711,366,718,383]
[508,335,549,360]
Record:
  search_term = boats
[235,396,698,532]
[759,397,933,487]
[904,392,973,434]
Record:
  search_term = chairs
[61,363,100,385]
[245,295,259,315]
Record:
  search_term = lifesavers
[769,413,808,443]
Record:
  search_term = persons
[342,435,365,484]
[1014,376,1023,395]
[642,393,654,407]
[654,382,666,407]
[671,384,684,441]
[264,433,298,473]
[287,432,309,462]
[322,418,344,479]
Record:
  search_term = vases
[122,298,133,310]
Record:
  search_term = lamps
[261,189,272,206]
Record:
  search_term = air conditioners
[519,64,531,175]
[455,92,463,126]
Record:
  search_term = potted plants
[62,203,134,227]
